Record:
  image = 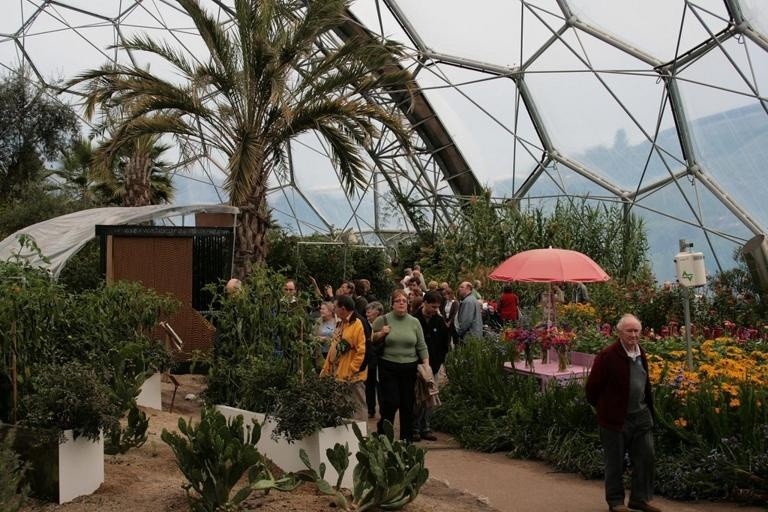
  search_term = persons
[585,314,662,512]
[568,282,590,304]
[542,283,565,304]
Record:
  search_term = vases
[507,345,574,371]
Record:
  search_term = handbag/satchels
[365,314,388,364]
[335,338,351,353]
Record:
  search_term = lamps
[340,227,357,245]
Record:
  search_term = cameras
[325,284,332,291]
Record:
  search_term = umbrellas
[488,245,611,365]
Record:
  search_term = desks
[503,357,593,394]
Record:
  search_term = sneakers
[627,500,662,512]
[367,412,438,442]
[609,504,644,512]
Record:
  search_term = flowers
[499,321,578,355]
